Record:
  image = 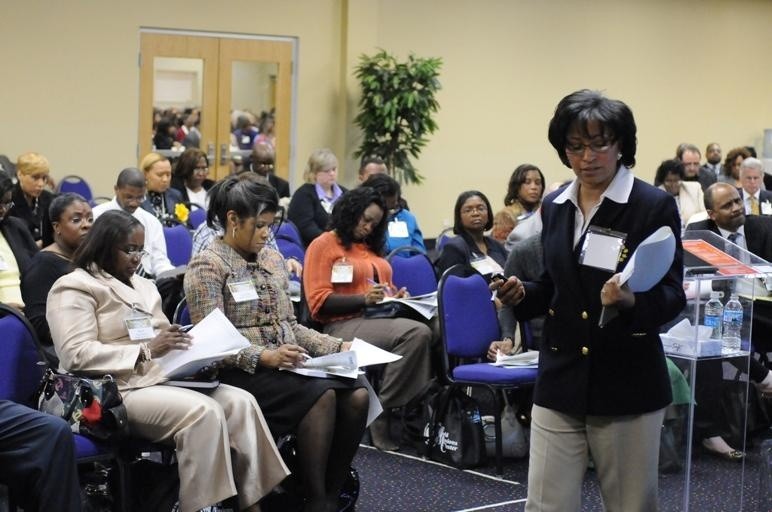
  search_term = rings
[601,290,607,295]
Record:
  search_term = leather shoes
[702,440,747,462]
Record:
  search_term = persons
[654,144,772,460]
[492,163,544,247]
[440,190,506,273]
[489,181,570,360]
[488,89,685,512]
[2,108,442,511]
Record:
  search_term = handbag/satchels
[36,369,131,444]
[720,379,770,444]
[659,424,682,475]
[273,437,358,511]
[421,386,527,466]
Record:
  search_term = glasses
[460,206,487,215]
[563,136,618,155]
[123,195,147,204]
[120,248,151,259]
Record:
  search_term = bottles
[704,292,743,354]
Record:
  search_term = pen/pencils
[364,277,390,295]
[499,338,507,353]
[175,323,194,333]
[300,352,312,359]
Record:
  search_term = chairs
[654,172,769,474]
[0,175,513,511]
[424,265,540,475]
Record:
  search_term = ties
[728,233,740,260]
[712,165,716,172]
[748,195,760,215]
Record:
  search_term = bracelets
[140,344,152,362]
